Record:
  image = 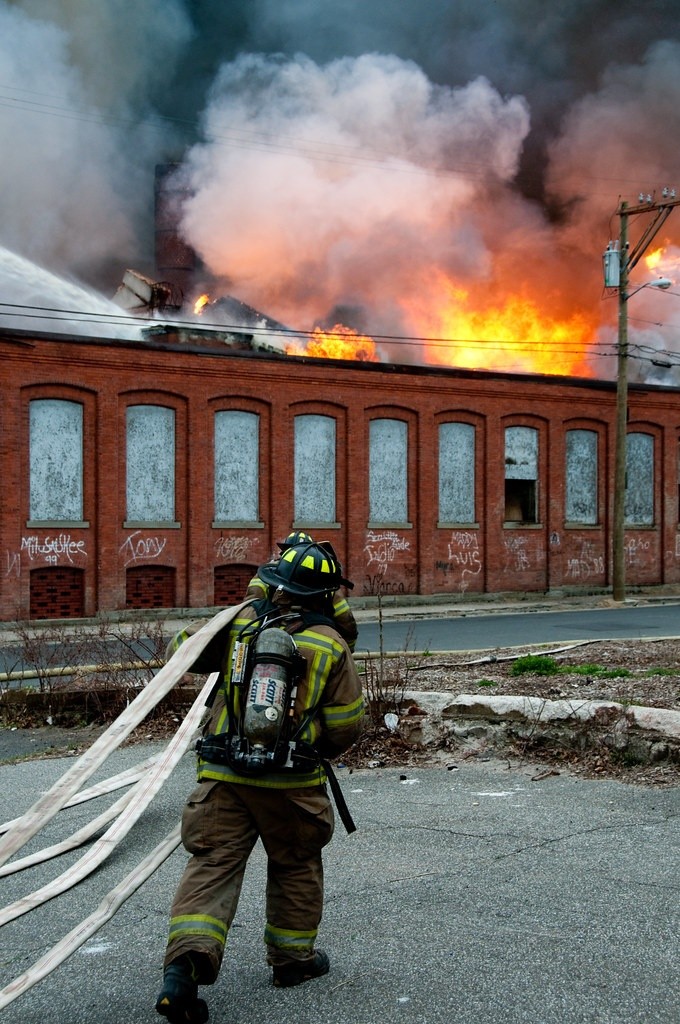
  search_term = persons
[154,530,363,1024]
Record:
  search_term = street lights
[611,276,673,602]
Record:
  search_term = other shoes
[273,946,330,987]
[156,968,209,1024]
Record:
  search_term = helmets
[258,542,341,595]
[277,531,313,552]
